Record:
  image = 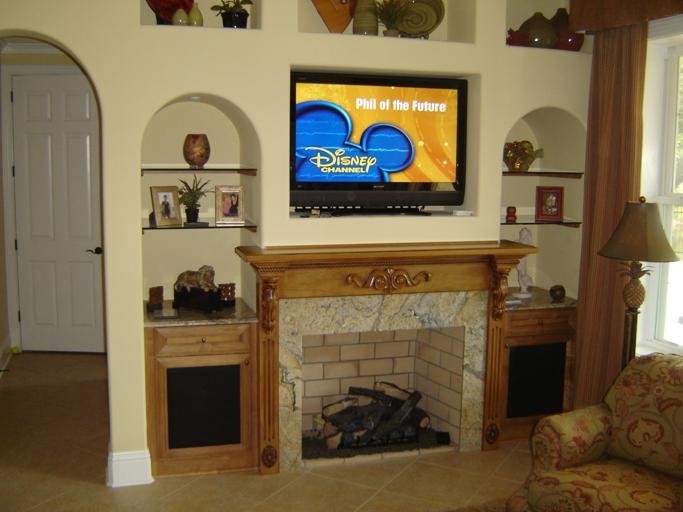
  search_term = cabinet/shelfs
[143,323,259,478]
[499,308,577,446]
[141,168,259,321]
[500,172,584,308]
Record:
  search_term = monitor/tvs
[290,68,468,216]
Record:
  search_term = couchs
[505,353,683,512]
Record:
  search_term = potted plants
[210,1,232,28]
[228,1,254,28]
[367,0,412,38]
[176,172,216,223]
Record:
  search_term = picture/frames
[215,186,245,224]
[535,186,564,221]
[151,186,182,225]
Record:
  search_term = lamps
[598,197,680,369]
